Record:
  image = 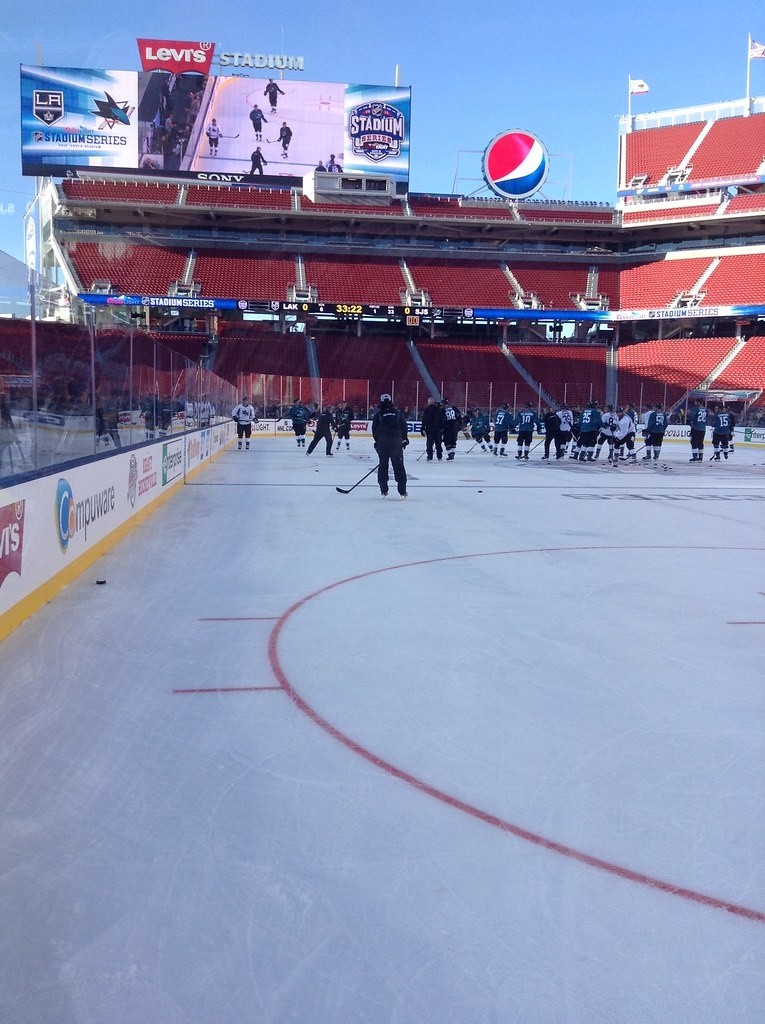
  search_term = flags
[630,80,648,93]
[750,40,765,59]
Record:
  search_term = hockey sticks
[416,438,435,462]
[521,438,544,458]
[331,418,341,441]
[709,449,722,461]
[619,444,646,461]
[335,464,380,494]
[235,417,283,422]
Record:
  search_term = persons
[232,396,259,452]
[228,397,765,468]
[0,390,232,450]
[372,394,409,501]
[250,147,267,175]
[264,78,284,114]
[249,105,268,141]
[142,74,209,170]
[278,122,292,158]
[688,398,709,463]
[306,405,339,458]
[205,119,222,157]
[315,154,343,173]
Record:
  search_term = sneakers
[237,441,242,450]
[305,449,311,457]
[437,454,442,462]
[325,449,333,457]
[246,442,250,449]
[345,442,351,452]
[335,441,341,451]
[426,454,433,462]
[723,446,729,460]
[478,445,663,467]
[698,451,703,463]
[295,438,301,447]
[378,485,389,500]
[397,486,408,501]
[729,443,735,453]
[689,450,698,463]
[302,438,306,447]
[713,448,721,463]
[446,452,456,462]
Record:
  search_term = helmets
[440,397,664,417]
[695,398,704,404]
[715,403,724,408]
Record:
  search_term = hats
[341,400,347,403]
[381,393,391,402]
[292,398,300,403]
[242,397,248,400]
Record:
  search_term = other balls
[477,491,483,493]
[466,431,490,454]
[314,469,320,473]
